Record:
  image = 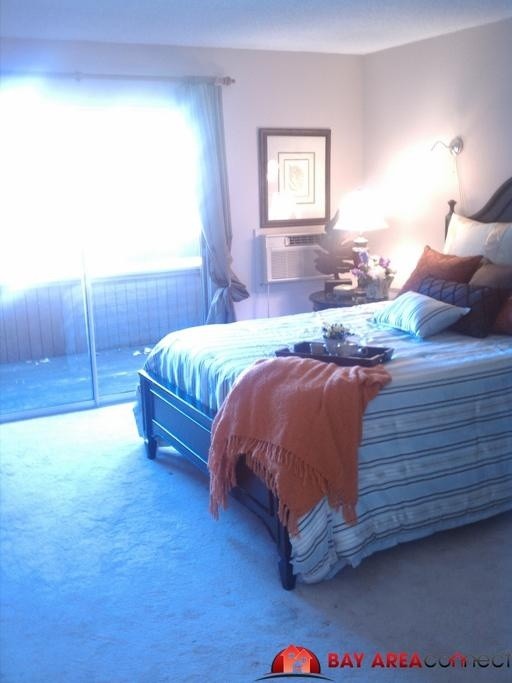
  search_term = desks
[308,288,401,312]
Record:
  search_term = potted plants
[312,210,358,295]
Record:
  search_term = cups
[308,339,367,356]
[351,291,367,304]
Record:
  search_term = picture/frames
[258,128,332,228]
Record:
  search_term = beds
[133,176,512,592]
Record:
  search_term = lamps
[422,112,463,157]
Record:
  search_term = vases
[359,277,394,299]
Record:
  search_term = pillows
[415,274,512,338]
[467,256,512,334]
[365,290,472,339]
[442,211,512,265]
[393,245,483,301]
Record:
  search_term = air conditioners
[258,233,335,285]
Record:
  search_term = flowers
[351,251,391,281]
